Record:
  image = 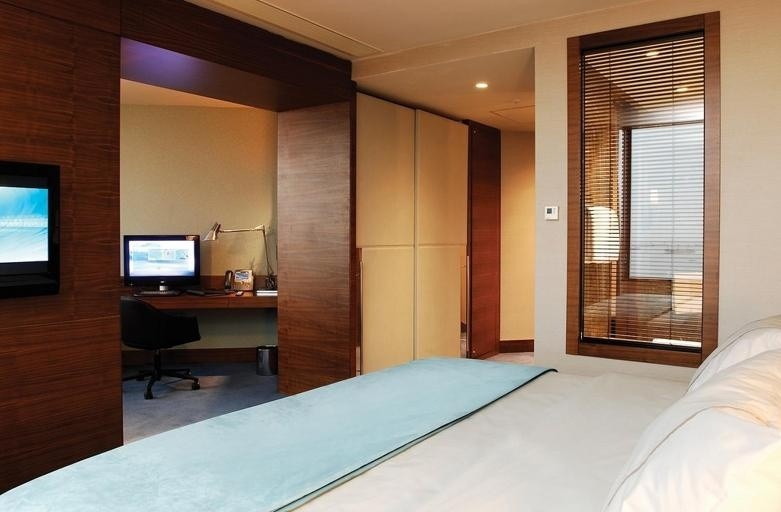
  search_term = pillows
[604,348,781,512]
[683,314,781,401]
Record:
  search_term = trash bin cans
[256,345,278,376]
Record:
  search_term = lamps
[200,218,275,291]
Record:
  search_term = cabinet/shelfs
[353,242,464,381]
[352,87,472,250]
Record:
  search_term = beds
[3,354,697,512]
[581,288,706,344]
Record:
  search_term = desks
[121,292,279,312]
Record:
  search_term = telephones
[225,269,254,290]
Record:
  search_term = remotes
[236,291,244,296]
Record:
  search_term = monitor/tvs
[0,160,62,299]
[123,235,200,297]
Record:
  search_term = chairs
[120,298,207,402]
